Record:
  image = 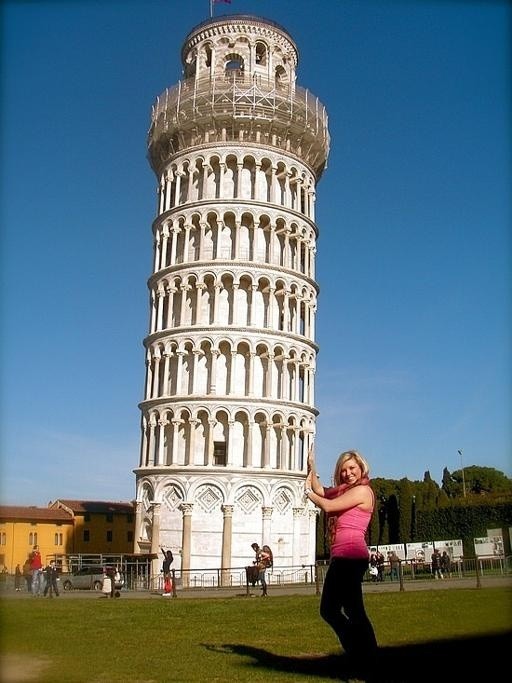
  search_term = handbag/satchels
[166,583,172,593]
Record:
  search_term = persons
[251,542,270,596]
[160,545,174,597]
[23,560,32,592]
[366,545,452,582]
[303,440,379,682]
[15,563,23,591]
[29,544,45,596]
[43,560,60,597]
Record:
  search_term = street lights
[457,446,466,500]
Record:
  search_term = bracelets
[304,488,313,494]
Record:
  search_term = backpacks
[263,545,273,568]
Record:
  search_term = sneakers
[163,594,170,596]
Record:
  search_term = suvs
[62,565,126,589]
